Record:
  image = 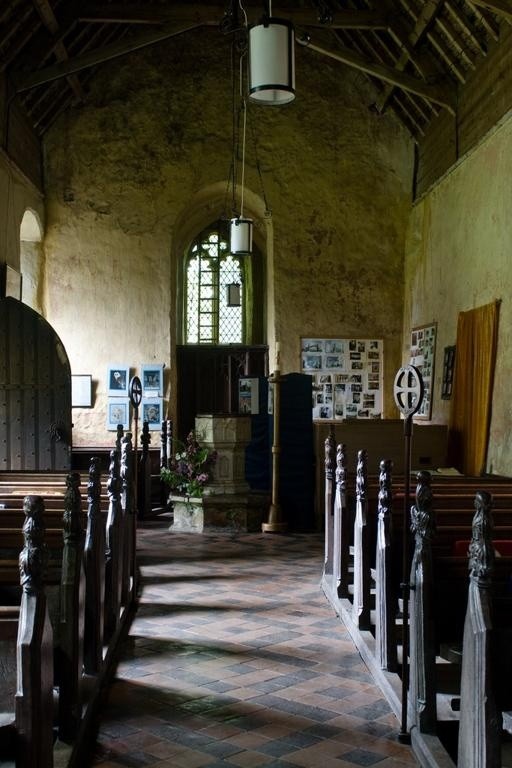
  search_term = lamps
[225,98,255,308]
[246,1,296,108]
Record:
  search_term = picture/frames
[71,363,165,432]
[298,322,438,424]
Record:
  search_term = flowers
[160,430,218,497]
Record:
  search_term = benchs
[0,425,140,767]
[323,422,512,767]
